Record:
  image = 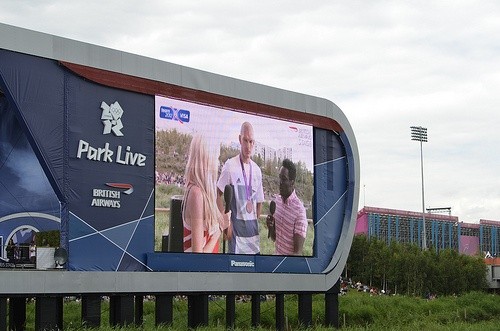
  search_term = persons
[265,158,308,257]
[216,120,265,255]
[5,277,402,305]
[5,231,54,266]
[180,130,233,254]
[156,168,187,189]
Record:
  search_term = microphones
[224,185,233,235]
[267,201,276,239]
